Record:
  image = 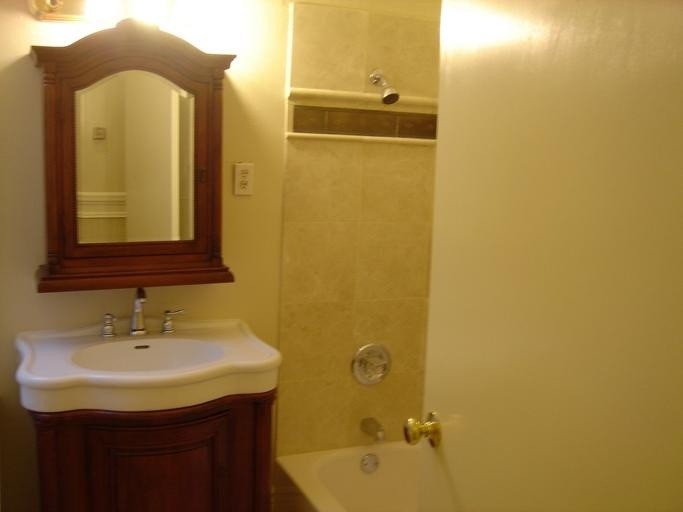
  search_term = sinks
[17,320,284,411]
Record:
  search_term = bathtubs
[276,439,465,512]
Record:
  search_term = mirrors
[29,19,236,290]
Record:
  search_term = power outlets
[235,163,255,196]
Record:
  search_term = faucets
[358,417,387,443]
[130,286,151,334]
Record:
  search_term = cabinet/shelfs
[29,390,276,511]
